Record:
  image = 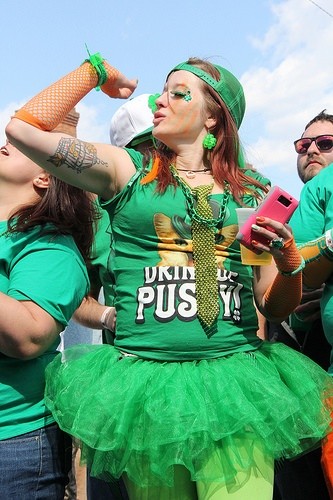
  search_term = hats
[109,93,156,150]
[50,107,80,139]
[166,58,245,131]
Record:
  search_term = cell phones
[236,185,299,254]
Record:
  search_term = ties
[189,183,220,329]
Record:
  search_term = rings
[270,238,284,250]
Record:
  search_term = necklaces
[176,168,211,179]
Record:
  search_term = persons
[0,52,333,500]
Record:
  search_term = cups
[236,208,272,266]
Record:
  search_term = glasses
[294,135,333,154]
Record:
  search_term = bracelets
[80,52,107,92]
[100,307,113,328]
[276,256,305,277]
